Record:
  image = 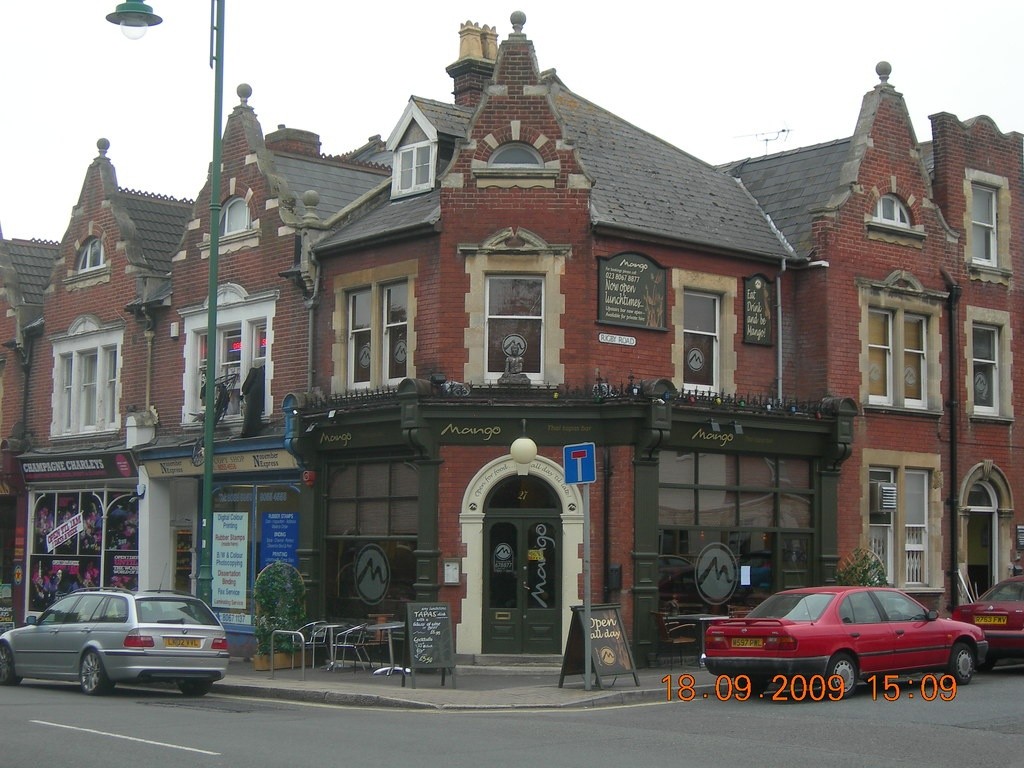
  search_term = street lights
[104,0,224,612]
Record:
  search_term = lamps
[951,285,962,310]
[509,419,538,464]
[432,374,447,385]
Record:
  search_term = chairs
[652,611,701,670]
[291,620,330,669]
[333,623,373,671]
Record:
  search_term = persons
[502,345,527,378]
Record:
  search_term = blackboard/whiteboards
[0,598,15,637]
[558,603,641,680]
[401,601,457,669]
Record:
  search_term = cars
[1,586,230,697]
[704,586,991,700]
[955,574,1023,672]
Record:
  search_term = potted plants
[250,562,314,673]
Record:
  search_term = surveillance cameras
[657,398,665,405]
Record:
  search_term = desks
[674,613,730,670]
[368,622,411,677]
[315,624,352,671]
